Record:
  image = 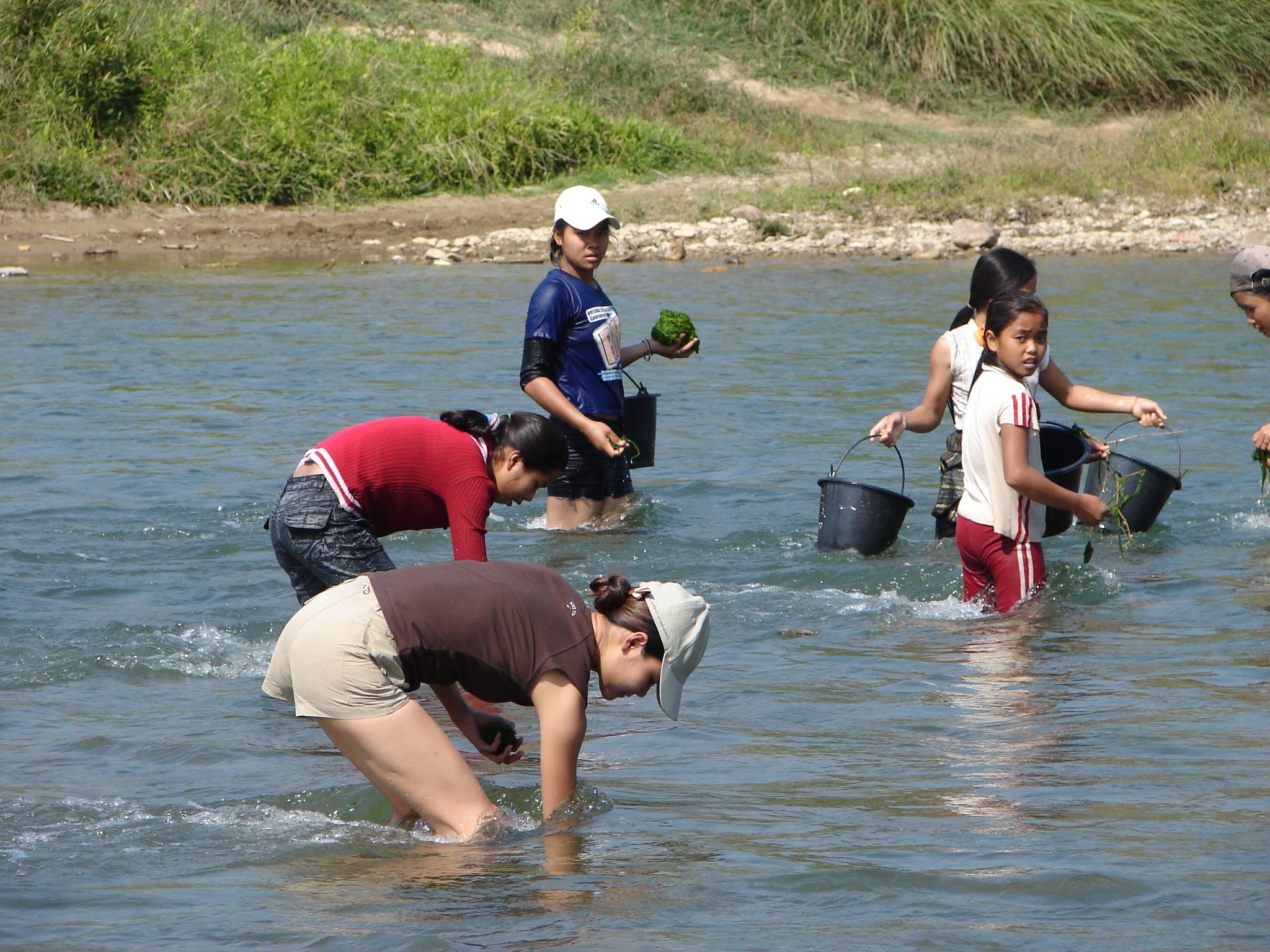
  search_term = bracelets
[642,339,653,362]
[1130,396,1139,413]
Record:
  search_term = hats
[1230,245,1270,293]
[637,580,711,723]
[553,185,619,231]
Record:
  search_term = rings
[678,350,681,355]
[1262,441,1268,446]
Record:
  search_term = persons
[520,185,701,529]
[264,409,569,607]
[870,245,1168,540]
[260,558,712,876]
[1230,243,1270,480]
[955,289,1113,614]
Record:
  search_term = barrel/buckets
[1035,421,1089,539]
[1081,417,1184,535]
[619,368,661,468]
[815,434,915,558]
